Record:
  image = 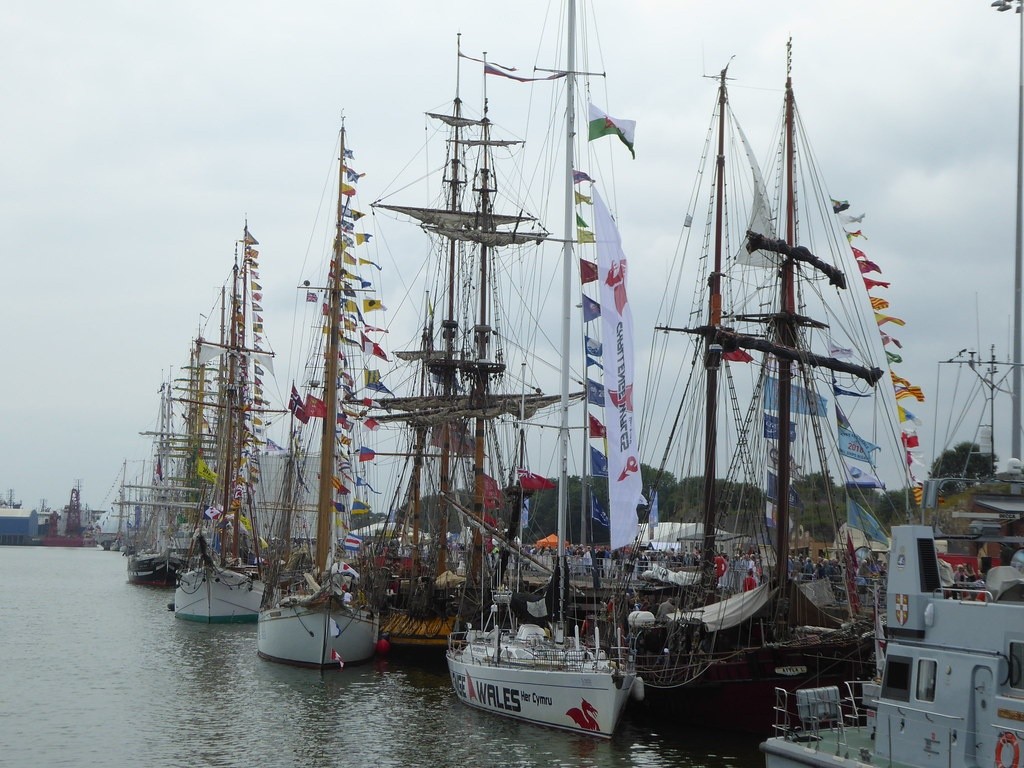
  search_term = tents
[536,533,568,548]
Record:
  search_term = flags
[844,496,890,547]
[289,385,310,425]
[763,374,827,533]
[197,247,265,538]
[153,459,163,485]
[649,487,659,527]
[587,100,637,160]
[830,195,932,509]
[482,474,505,510]
[572,166,614,526]
[330,616,345,640]
[522,496,529,528]
[302,391,327,418]
[518,468,556,491]
[331,147,389,582]
[332,651,345,668]
[306,292,317,302]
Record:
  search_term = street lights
[990,1,1024,464]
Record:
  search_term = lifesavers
[995,731,1019,768]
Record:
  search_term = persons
[606,593,616,617]
[955,563,983,600]
[360,536,889,608]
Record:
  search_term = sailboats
[93,2,1024,768]
